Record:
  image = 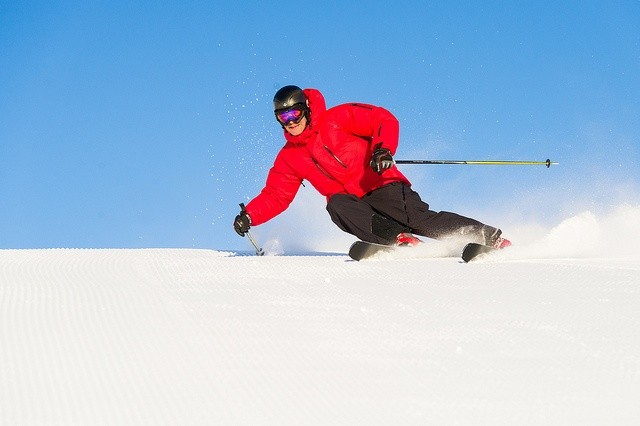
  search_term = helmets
[274,85,306,111]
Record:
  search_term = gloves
[370,148,393,174]
[233,203,250,236]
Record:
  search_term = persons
[232,83,514,250]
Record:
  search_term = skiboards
[346,240,501,263]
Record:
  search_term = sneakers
[394,233,423,246]
[493,237,512,249]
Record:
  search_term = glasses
[276,106,305,125]
